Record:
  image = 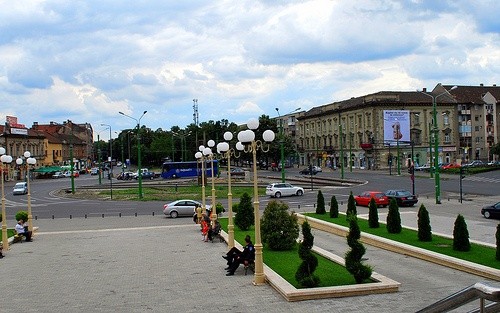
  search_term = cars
[257,159,292,168]
[306,165,322,172]
[299,168,317,175]
[226,168,245,176]
[52,159,128,178]
[438,159,494,169]
[353,190,388,208]
[414,164,436,171]
[116,168,155,180]
[163,199,211,218]
[385,189,418,207]
[481,201,500,220]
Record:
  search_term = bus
[160,159,218,179]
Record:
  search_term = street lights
[16,150,37,231]
[416,85,458,204]
[216,131,244,251]
[195,145,209,214]
[202,139,217,230]
[53,121,75,194]
[119,110,148,199]
[238,118,275,289]
[275,107,302,182]
[0,146,14,253]
[100,123,113,199]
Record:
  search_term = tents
[31,163,70,181]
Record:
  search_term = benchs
[211,226,223,242]
[244,262,255,274]
[13,230,24,242]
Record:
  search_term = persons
[193,204,221,244]
[15,219,33,243]
[221,235,255,276]
[122,173,130,181]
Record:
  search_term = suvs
[13,182,28,195]
[265,182,304,197]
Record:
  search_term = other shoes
[227,270,235,272]
[226,273,234,276]
[225,267,231,270]
[222,256,229,261]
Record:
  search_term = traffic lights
[388,155,392,165]
[408,167,412,174]
